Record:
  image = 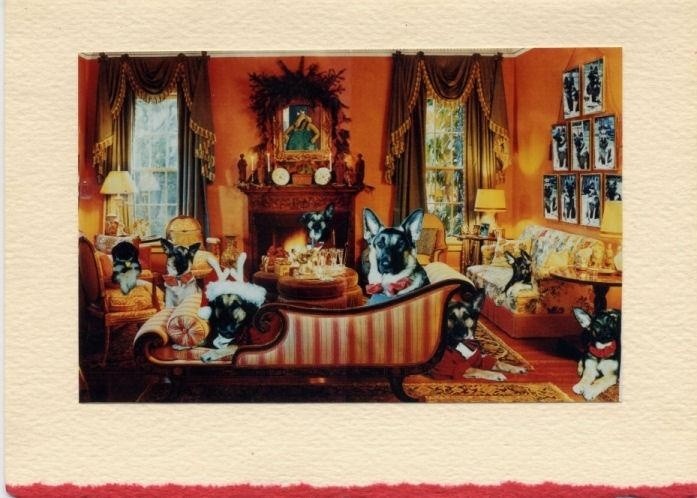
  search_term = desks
[466,235,496,264]
[252,267,364,311]
[551,272,622,312]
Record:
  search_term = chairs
[79,215,219,370]
[361,212,448,274]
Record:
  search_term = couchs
[465,224,605,339]
[132,262,477,403]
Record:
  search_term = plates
[314,167,332,186]
[272,167,290,186]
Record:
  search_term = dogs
[111,240,141,294]
[501,250,533,293]
[543,67,623,226]
[297,202,336,249]
[458,285,486,339]
[362,207,431,297]
[571,306,621,401]
[429,301,527,383]
[160,237,202,307]
[197,252,266,363]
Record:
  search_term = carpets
[132,383,574,403]
[79,295,534,372]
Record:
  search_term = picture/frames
[542,56,623,230]
[273,97,329,161]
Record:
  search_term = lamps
[473,188,506,233]
[99,171,139,236]
[601,201,623,272]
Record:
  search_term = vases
[221,236,242,267]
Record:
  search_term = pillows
[417,228,438,255]
[492,238,575,274]
[166,294,212,347]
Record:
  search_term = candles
[267,152,271,172]
[251,155,254,168]
[329,150,332,171]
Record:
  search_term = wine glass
[311,248,344,280]
